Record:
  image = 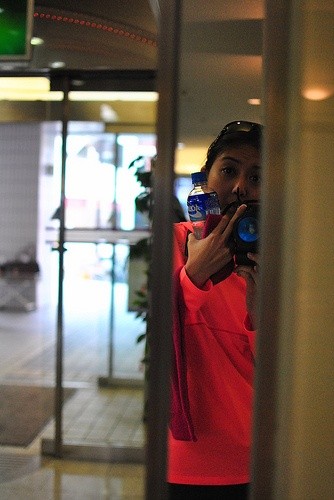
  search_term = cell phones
[202,214,223,239]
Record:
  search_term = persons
[168,120,271,499]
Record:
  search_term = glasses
[209,121,265,151]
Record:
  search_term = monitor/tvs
[0,0,35,61]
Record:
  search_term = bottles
[187,172,221,240]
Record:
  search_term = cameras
[225,200,260,266]
